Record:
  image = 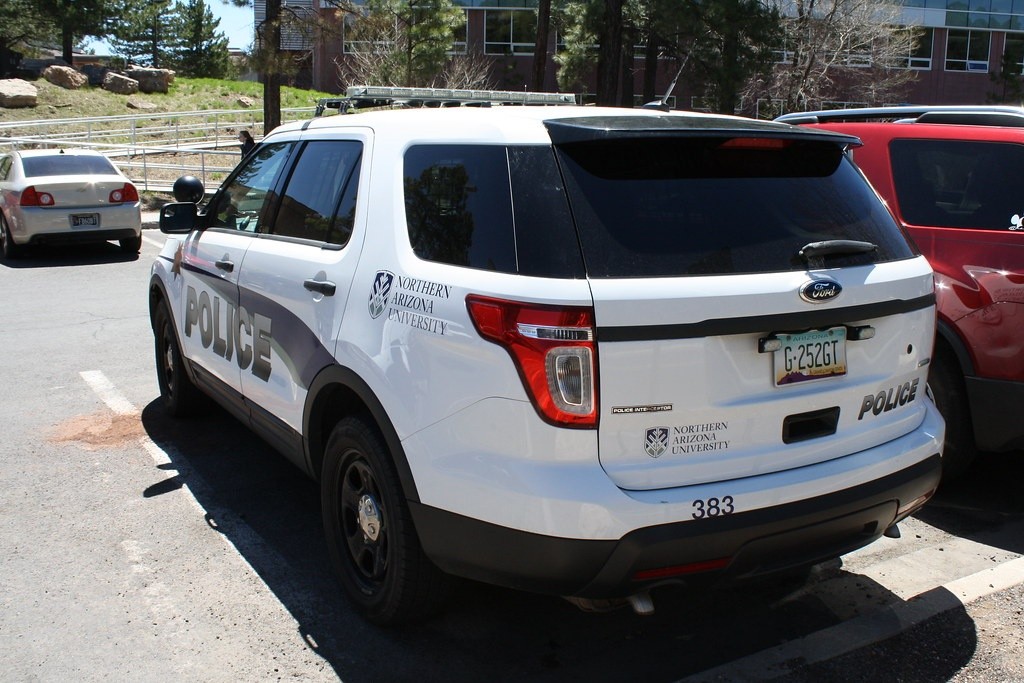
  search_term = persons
[238,131,255,160]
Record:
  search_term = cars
[0,149,142,258]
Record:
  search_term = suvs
[725,106,1024,475]
[146,86,948,621]
